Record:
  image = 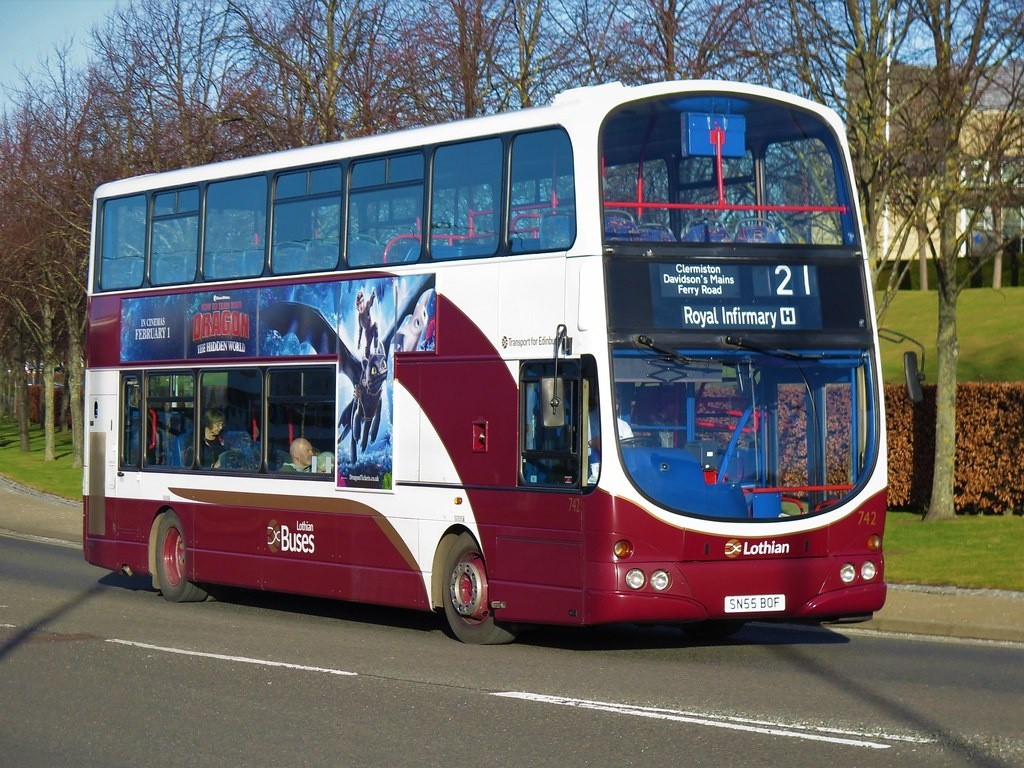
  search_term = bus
[83,81,925,646]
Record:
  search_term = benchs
[126,409,293,471]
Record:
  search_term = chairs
[102,207,779,289]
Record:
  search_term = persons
[186,409,227,468]
[277,438,319,473]
[588,386,633,483]
